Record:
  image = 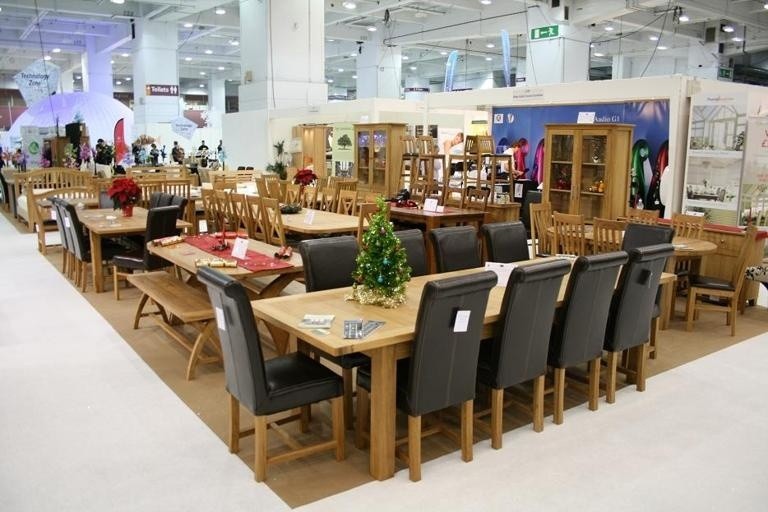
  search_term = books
[299,314,336,330]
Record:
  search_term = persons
[500,143,524,180]
[135,138,223,166]
[1,146,24,172]
[94,139,113,166]
[440,132,463,153]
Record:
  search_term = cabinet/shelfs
[543,120,636,223]
[290,122,329,178]
[353,122,408,197]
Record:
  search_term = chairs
[129,136,677,483]
[529,202,767,337]
[1,135,263,302]
